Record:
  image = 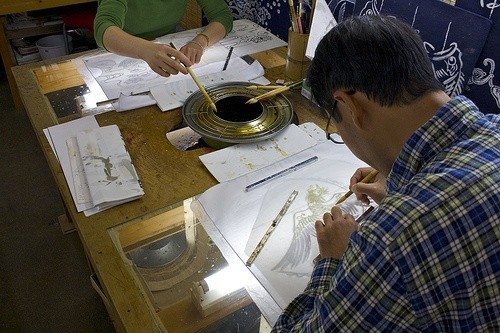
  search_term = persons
[272,15,500,333]
[94,0,233,77]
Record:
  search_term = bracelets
[191,41,203,48]
[200,34,209,46]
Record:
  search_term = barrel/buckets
[36,35,72,60]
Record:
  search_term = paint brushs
[289,0,299,33]
[246,85,302,90]
[170,42,217,113]
[245,78,303,104]
[334,168,378,205]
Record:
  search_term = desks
[11,19,381,333]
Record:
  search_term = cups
[285,26,311,81]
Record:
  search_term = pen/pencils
[297,0,307,34]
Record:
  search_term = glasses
[326,89,356,144]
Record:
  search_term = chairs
[63,13,96,55]
